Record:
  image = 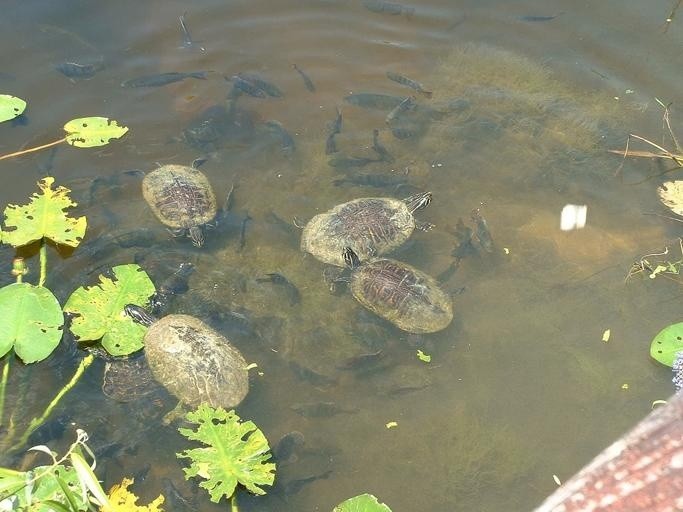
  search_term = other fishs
[0,58,495,512]
